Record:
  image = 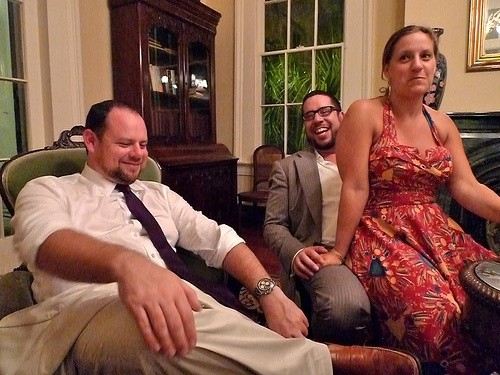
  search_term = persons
[316,25,500,375]
[11,101,421,375]
[263,90,371,346]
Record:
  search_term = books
[153,105,178,137]
[190,111,211,139]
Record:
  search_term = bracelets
[332,248,346,262]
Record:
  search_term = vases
[424,28,447,111]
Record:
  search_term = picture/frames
[466,0,500,72]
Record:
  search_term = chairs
[0,126,163,216]
[239,145,284,231]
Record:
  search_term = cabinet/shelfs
[108,0,241,231]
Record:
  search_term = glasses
[302,105,338,122]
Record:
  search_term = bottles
[422,28,448,110]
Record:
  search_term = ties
[114,182,248,312]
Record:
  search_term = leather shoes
[325,340,423,374]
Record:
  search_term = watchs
[254,278,279,302]
